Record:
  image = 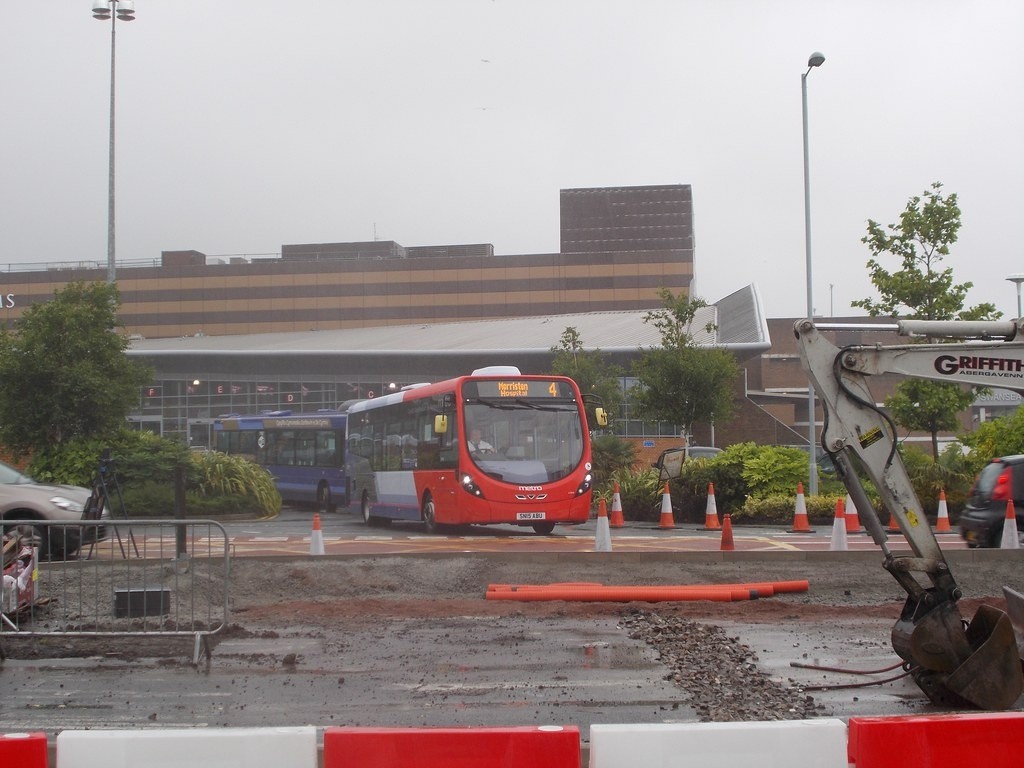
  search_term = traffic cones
[650,481,682,529]
[609,483,632,528]
[932,489,957,534]
[845,492,868,534]
[309,514,326,555]
[1000,499,1020,550]
[885,511,902,534]
[594,496,612,552]
[784,483,816,533]
[720,514,736,550]
[829,499,847,551]
[695,482,722,531]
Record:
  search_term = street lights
[1005,275,1024,317]
[94,0,136,334]
[801,51,826,499]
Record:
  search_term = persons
[467,429,495,454]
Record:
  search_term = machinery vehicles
[793,316,1024,712]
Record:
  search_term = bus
[344,366,608,535]
[211,399,363,513]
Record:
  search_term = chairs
[347,432,439,470]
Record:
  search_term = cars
[651,446,724,469]
[0,462,109,569]
[958,455,1024,549]
